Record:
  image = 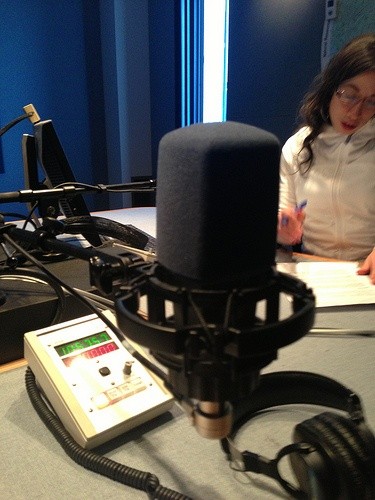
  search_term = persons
[277,34,375,287]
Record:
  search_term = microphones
[155,122,282,293]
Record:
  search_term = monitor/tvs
[22,119,101,246]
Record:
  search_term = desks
[0,207,375,500]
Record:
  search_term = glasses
[335,87,375,110]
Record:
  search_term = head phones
[220,370,375,500]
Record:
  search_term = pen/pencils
[282,200,307,226]
